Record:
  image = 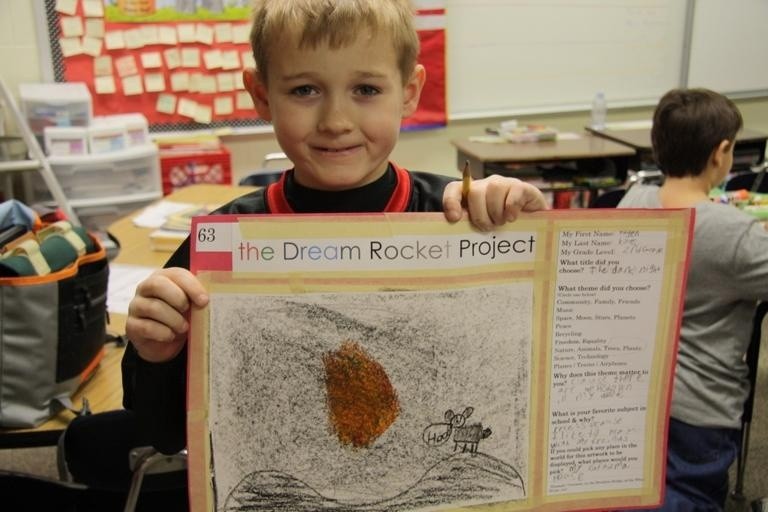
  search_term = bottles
[590,91,606,131]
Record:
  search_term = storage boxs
[43,125,88,158]
[90,110,150,156]
[38,147,163,233]
[16,79,93,136]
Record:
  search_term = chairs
[55,409,188,511]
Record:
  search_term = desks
[450,132,636,190]
[0,182,265,449]
[585,119,768,176]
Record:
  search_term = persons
[123,1,548,455]
[614,86,768,511]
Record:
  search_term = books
[149,203,222,252]
[505,123,557,144]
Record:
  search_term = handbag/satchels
[0,223,108,429]
